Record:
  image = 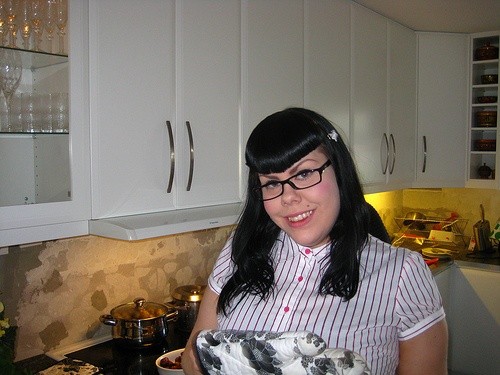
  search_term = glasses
[253,159,332,201]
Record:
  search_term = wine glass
[0,0,68,56]
[0,48,23,133]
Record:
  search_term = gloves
[196,329,372,375]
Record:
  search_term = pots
[99,284,206,345]
[403,212,441,230]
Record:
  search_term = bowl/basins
[481,74,498,85]
[474,139,496,151]
[474,42,498,61]
[476,111,497,127]
[476,96,498,103]
[155,348,185,375]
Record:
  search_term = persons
[181,107,448,375]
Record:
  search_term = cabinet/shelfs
[0,0,500,246]
[434,266,500,375]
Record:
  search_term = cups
[1,93,69,132]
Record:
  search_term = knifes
[479,204,485,223]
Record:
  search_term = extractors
[88,201,246,241]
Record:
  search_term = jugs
[478,162,493,176]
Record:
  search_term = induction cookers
[45,313,194,375]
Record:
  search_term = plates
[422,248,453,260]
